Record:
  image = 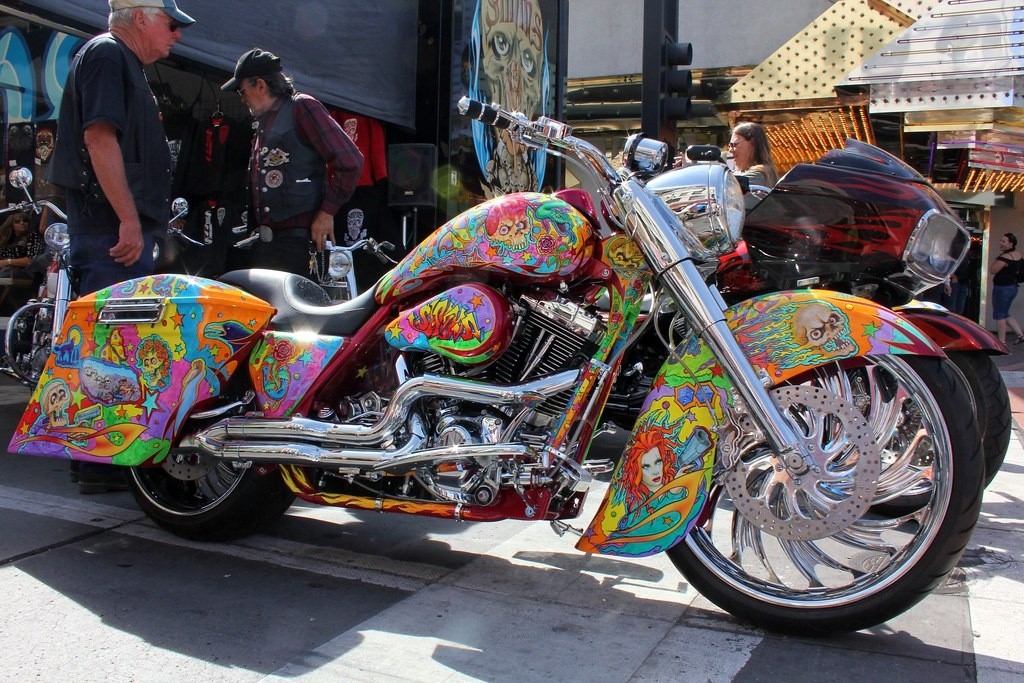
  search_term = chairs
[0,266,34,315]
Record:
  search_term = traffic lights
[665,42,692,120]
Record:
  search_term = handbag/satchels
[1017,259,1024,283]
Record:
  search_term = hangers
[210,99,224,118]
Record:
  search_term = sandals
[1013,335,1024,345]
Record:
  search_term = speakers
[6,119,58,206]
[387,142,437,208]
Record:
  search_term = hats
[108,0,196,29]
[221,48,283,91]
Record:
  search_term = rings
[323,236,327,237]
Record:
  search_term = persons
[51,0,195,293]
[0,213,46,278]
[222,48,363,285]
[920,256,980,316]
[26,207,63,295]
[989,233,1024,347]
[674,122,779,189]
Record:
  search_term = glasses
[729,139,746,148]
[152,13,178,32]
[237,82,256,97]
[13,217,29,224]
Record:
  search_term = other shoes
[70,460,80,482]
[79,469,130,493]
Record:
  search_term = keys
[308,259,315,274]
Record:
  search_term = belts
[259,225,310,243]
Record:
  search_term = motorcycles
[0,167,398,397]
[595,136,1013,519]
[7,95,986,637]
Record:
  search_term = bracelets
[8,259,12,264]
